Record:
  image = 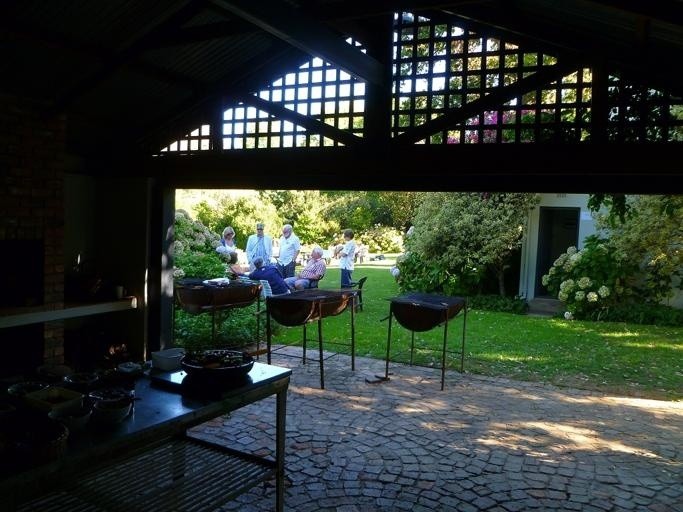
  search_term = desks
[0,360,292,511]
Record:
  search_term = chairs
[341,277,367,313]
[259,280,291,300]
[309,274,324,288]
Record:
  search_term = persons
[246,223,273,270]
[276,224,300,278]
[248,257,290,295]
[216,227,235,253]
[284,246,326,291]
[227,252,244,276]
[335,230,356,288]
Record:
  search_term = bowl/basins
[8,382,41,398]
[65,373,99,393]
[93,400,132,423]
